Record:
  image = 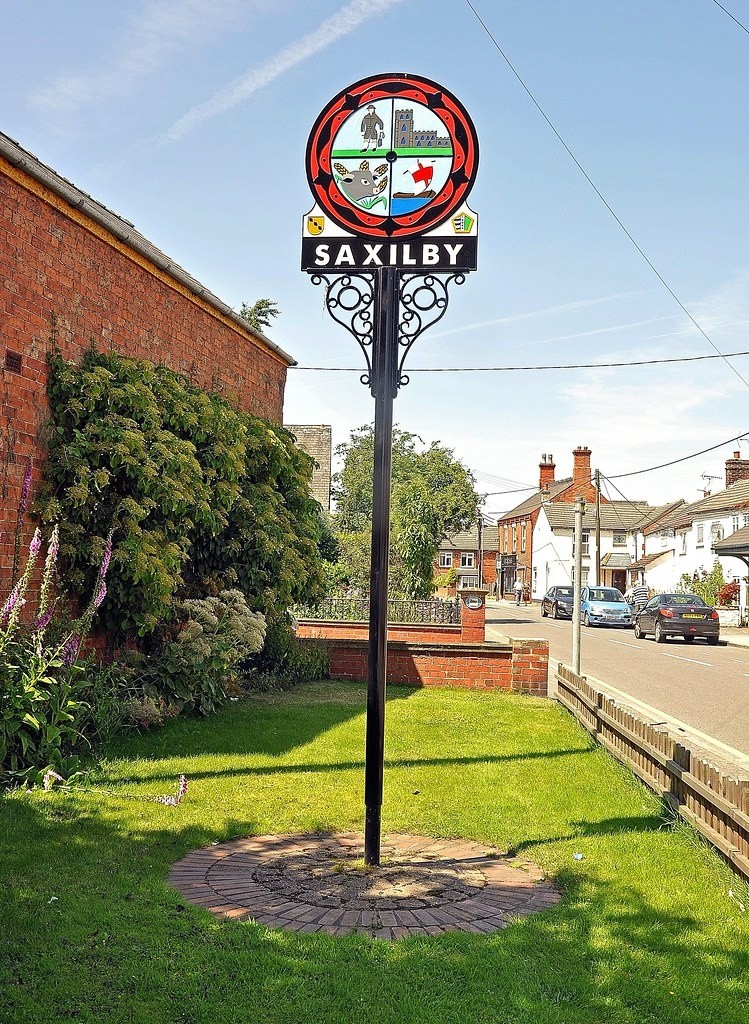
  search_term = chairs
[605,592,610,599]
[589,591,594,598]
[687,600,695,604]
[558,591,562,596]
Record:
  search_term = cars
[579,586,634,628]
[634,593,719,645]
[541,584,577,621]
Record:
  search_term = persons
[514,577,530,606]
[624,580,649,611]
